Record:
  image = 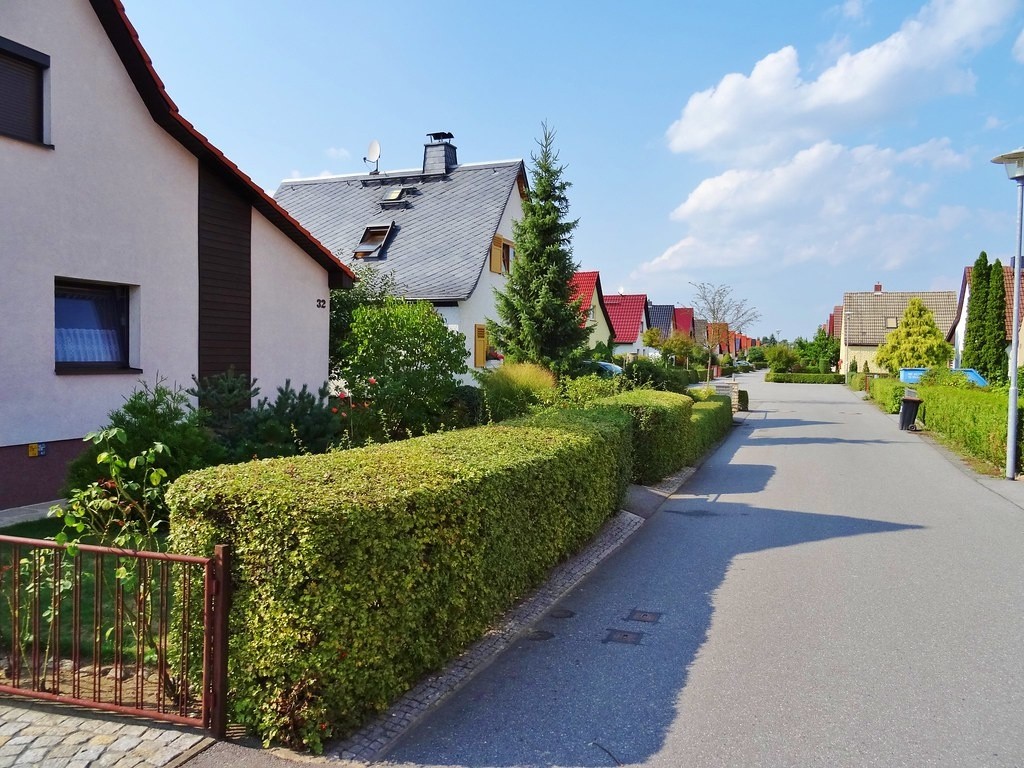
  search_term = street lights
[776,329,782,345]
[989,147,1023,481]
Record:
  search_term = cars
[570,360,623,380]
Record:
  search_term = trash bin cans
[898,397,924,430]
